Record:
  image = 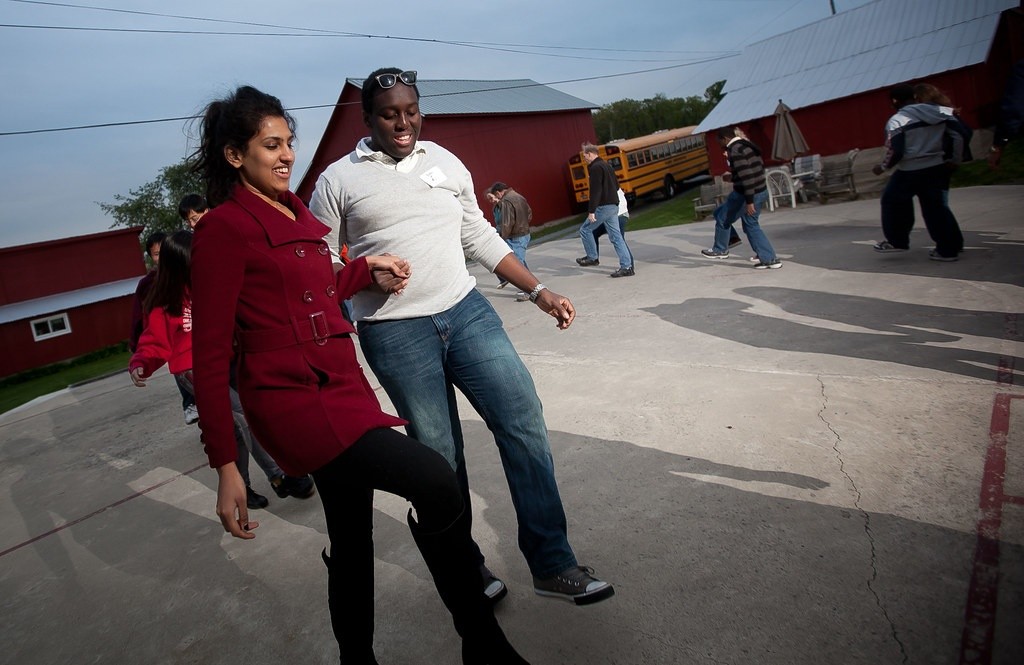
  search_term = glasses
[361,70,418,96]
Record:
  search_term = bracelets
[528,284,548,303]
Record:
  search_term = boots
[322,543,379,665]
[407,502,534,665]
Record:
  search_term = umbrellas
[770,103,811,161]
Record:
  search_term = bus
[565,123,710,211]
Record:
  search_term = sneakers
[701,248,729,260]
[482,563,507,600]
[531,564,615,605]
[754,255,783,270]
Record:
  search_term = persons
[700,126,782,269]
[127,68,635,665]
[190,85,531,665]
[870,83,973,262]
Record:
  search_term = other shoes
[576,256,599,266]
[246,485,269,509]
[874,241,910,252]
[271,479,287,498]
[611,267,634,277]
[497,279,508,288]
[727,240,742,248]
[750,254,760,261]
[183,404,199,424]
[925,246,958,261]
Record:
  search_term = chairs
[693,184,723,218]
[766,154,858,212]
